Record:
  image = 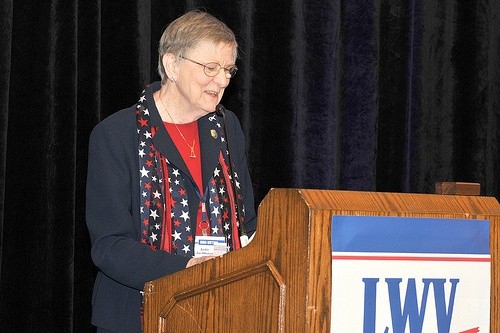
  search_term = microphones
[215,104,248,247]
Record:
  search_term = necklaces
[159,89,197,158]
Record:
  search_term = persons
[85,11,257,333]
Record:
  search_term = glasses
[179,54,238,78]
